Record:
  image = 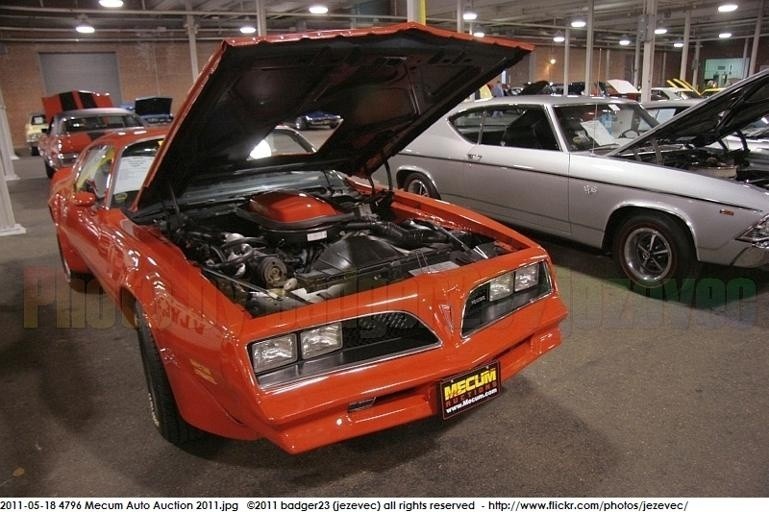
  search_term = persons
[493,81,506,118]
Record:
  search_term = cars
[46,21,569,456]
[280,114,343,132]
[24,90,172,180]
[372,67,769,299]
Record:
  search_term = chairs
[461,119,587,150]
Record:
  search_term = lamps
[75,0,738,48]
